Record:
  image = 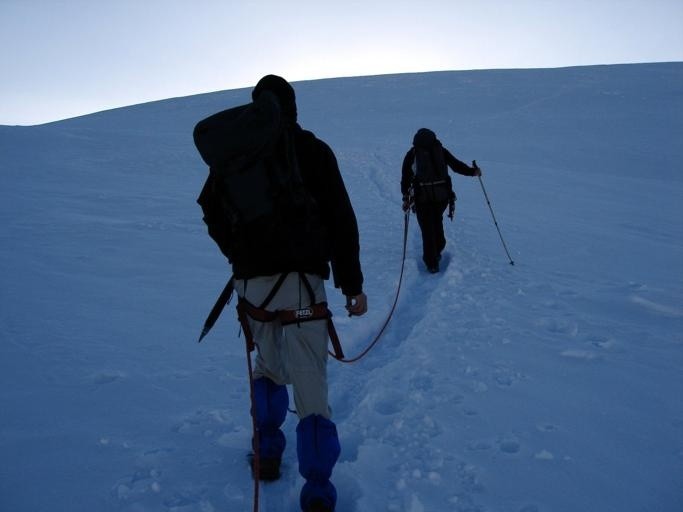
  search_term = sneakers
[422,254,445,274]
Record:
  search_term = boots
[251,374,288,483]
[296,414,341,511]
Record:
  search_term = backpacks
[192,101,301,263]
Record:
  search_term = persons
[194,73,368,511]
[397,126,481,274]
